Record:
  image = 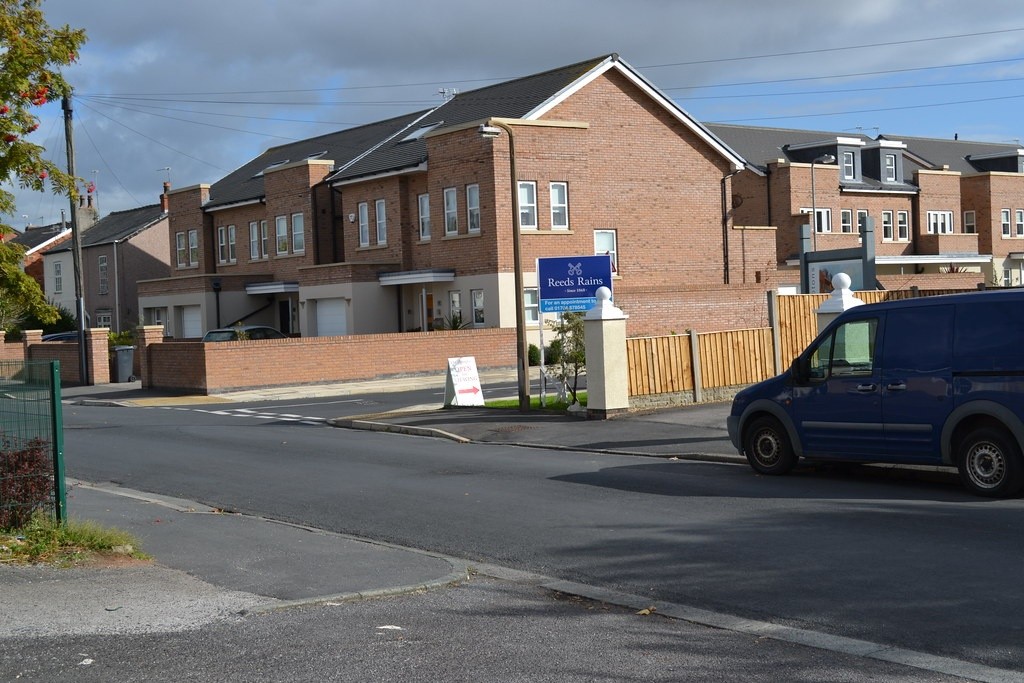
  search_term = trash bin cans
[110,344,137,382]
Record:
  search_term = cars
[42,330,78,343]
[201,326,288,343]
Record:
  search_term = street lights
[811,153,836,253]
[479,119,536,411]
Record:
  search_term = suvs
[725,288,1024,499]
